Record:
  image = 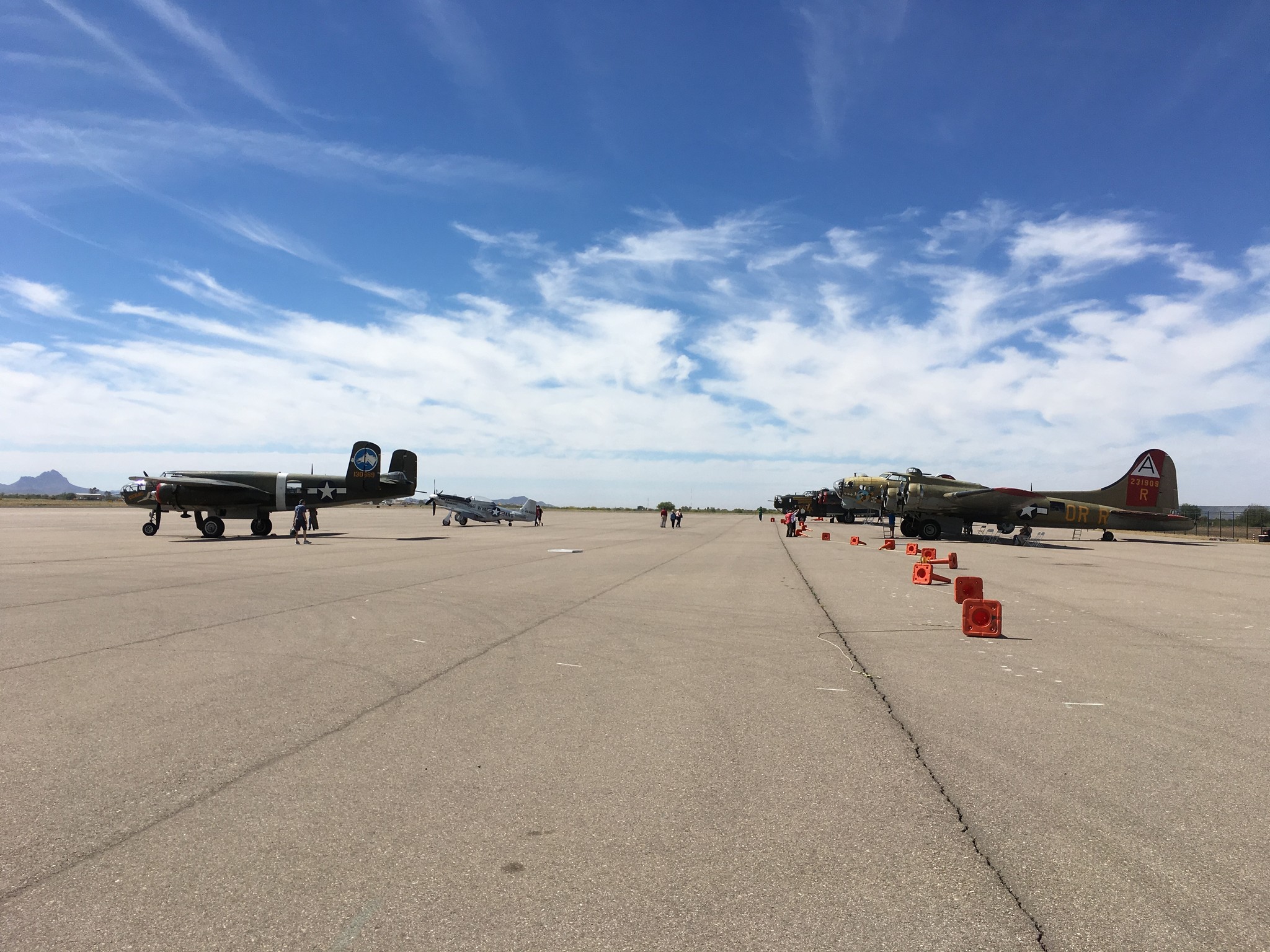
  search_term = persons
[307,507,318,531]
[660,507,668,528]
[967,520,973,535]
[1009,523,1033,545]
[789,509,799,537]
[674,509,682,528]
[534,505,540,526]
[963,519,969,535]
[292,499,312,545]
[758,506,763,522]
[784,509,797,537]
[881,510,896,538]
[670,510,676,529]
[537,505,544,526]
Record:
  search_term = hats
[679,508,681,510]
[299,499,304,504]
[786,509,790,512]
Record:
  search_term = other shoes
[660,525,682,529]
[964,533,973,536]
[307,529,310,530]
[760,520,762,522]
[1009,543,1025,546]
[313,529,315,530]
[535,523,543,526]
[786,534,798,537]
[890,536,894,538]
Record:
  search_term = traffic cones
[953,575,1004,641]
[770,516,958,587]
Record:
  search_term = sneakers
[303,540,312,544]
[295,540,300,544]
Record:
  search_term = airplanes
[425,479,538,526]
[832,445,1197,542]
[111,441,427,536]
[767,487,882,524]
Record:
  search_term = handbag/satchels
[290,527,296,536]
